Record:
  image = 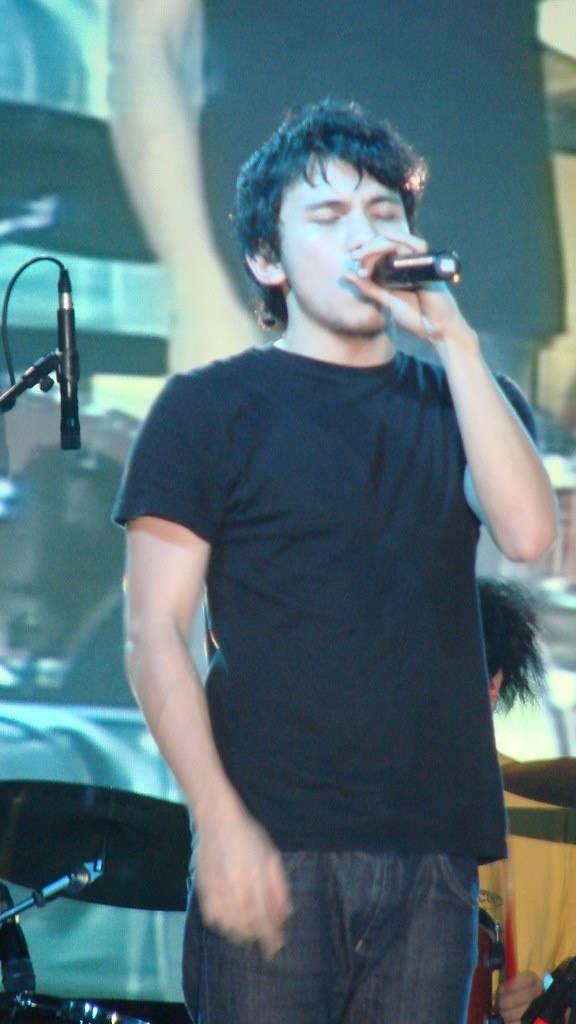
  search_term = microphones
[348,248,462,282]
[58,269,82,451]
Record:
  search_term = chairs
[0,779,192,1024]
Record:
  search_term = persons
[111,102,559,1023]
[114,0,567,447]
[477,579,576,1024]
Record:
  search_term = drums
[7,987,192,1023]
[463,905,507,1024]
[2,441,134,663]
[517,453,576,660]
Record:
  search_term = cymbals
[499,756,576,810]
[0,94,161,264]
[1,776,195,915]
